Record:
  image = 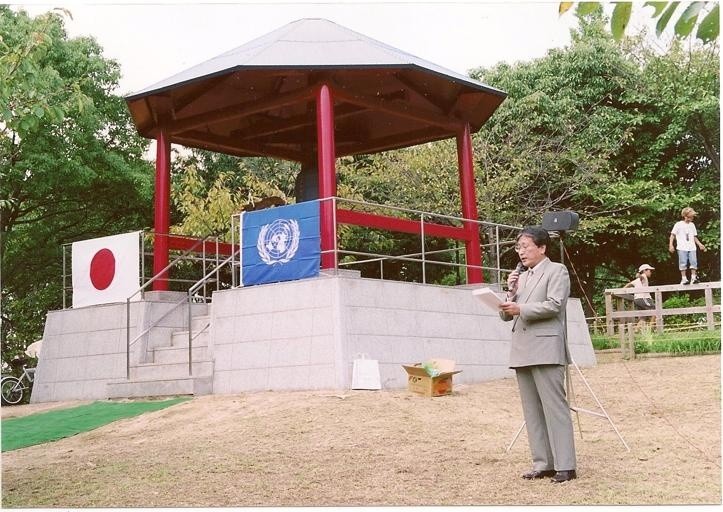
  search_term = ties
[526,272,532,286]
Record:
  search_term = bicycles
[1,356,39,408]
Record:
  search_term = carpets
[1,398,191,453]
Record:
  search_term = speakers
[542,212,579,231]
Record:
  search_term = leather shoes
[550,470,576,483]
[522,471,555,480]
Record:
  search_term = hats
[638,264,655,273]
[680,207,696,218]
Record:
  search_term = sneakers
[691,278,699,284]
[680,279,689,284]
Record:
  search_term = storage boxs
[401,362,464,398]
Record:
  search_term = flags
[71,230,143,311]
[239,200,322,286]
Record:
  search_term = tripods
[496,233,630,457]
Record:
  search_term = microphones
[508,261,522,293]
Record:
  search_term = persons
[624,263,657,333]
[668,206,706,285]
[498,227,577,484]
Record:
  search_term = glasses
[514,245,528,253]
